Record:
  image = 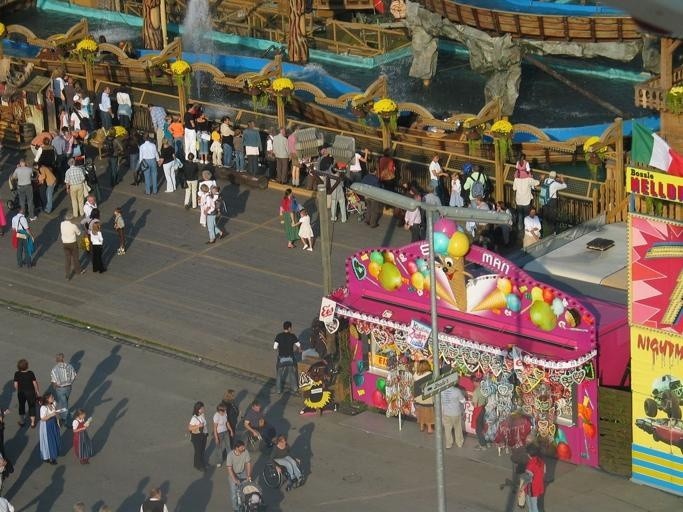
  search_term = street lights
[347,182,512,512]
[291,127,355,373]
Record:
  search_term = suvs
[643,374,683,417]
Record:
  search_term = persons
[187,389,303,512]
[413,360,436,434]
[265,125,397,251]
[400,152,568,253]
[273,321,301,394]
[135,100,262,242]
[1,87,143,280]
[13,352,94,465]
[440,386,466,449]
[140,487,169,512]
[517,443,547,512]
[470,375,491,451]
[50,35,137,64]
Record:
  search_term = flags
[631,118,683,177]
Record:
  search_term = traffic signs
[421,371,458,399]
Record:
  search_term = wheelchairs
[239,416,272,450]
[262,441,304,488]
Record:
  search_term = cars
[636,417,656,434]
[651,418,683,448]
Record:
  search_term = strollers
[235,480,267,511]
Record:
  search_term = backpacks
[84,166,97,185]
[469,172,484,198]
[539,180,553,206]
